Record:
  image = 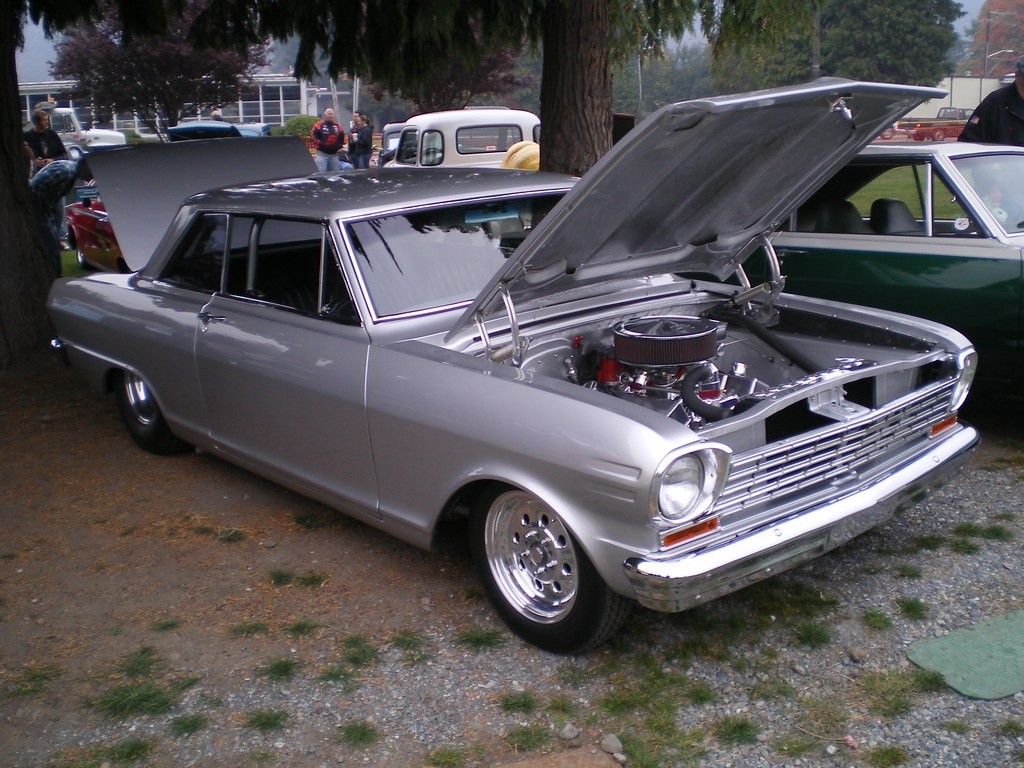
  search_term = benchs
[213,242,348,313]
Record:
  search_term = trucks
[22,98,127,148]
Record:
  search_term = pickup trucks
[897,108,974,141]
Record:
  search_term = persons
[21,110,95,279]
[503,139,540,170]
[958,57,1024,233]
[211,111,224,123]
[348,109,373,169]
[311,108,345,172]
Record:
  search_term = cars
[66,199,130,274]
[166,119,272,143]
[42,78,980,654]
[338,105,542,171]
[676,144,1024,438]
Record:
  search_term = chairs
[814,200,878,234]
[871,198,922,233]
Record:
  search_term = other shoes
[60,242,70,251]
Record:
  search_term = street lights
[985,49,1014,79]
